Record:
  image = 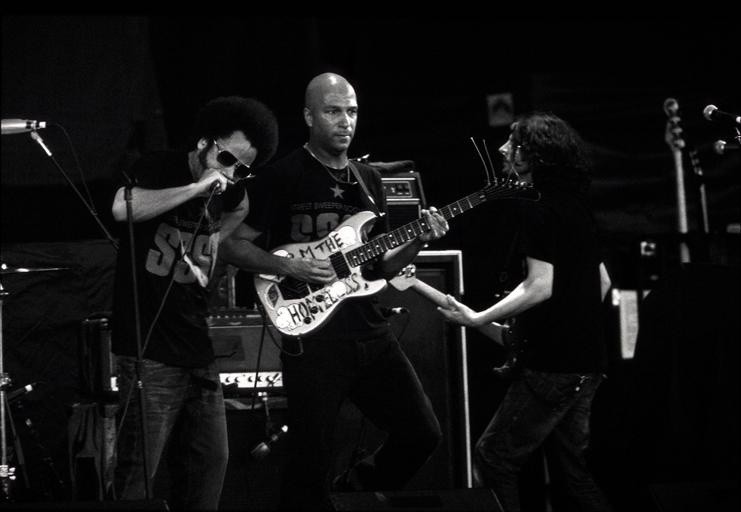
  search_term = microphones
[701,103,741,126]
[208,181,222,195]
[8,381,38,404]
[712,139,741,155]
[0,118,58,137]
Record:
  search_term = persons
[108,94,279,511]
[436,110,612,512]
[219,71,450,511]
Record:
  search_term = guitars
[252,179,533,336]
[388,263,536,378]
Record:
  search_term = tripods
[0,295,31,500]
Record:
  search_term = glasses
[209,140,254,181]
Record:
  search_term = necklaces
[304,143,350,185]
